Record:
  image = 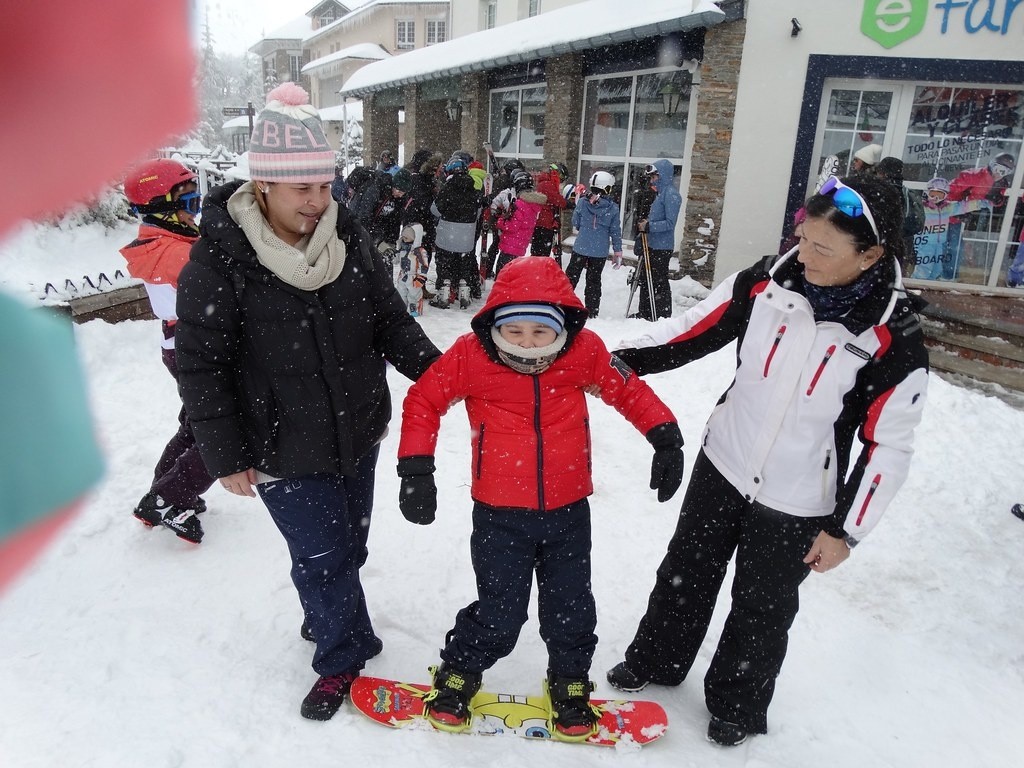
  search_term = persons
[853,144,883,174]
[909,151,1024,288]
[396,255,684,735]
[629,159,683,321]
[485,159,623,319]
[175,80,462,721]
[119,156,219,544]
[331,148,487,310]
[395,222,428,317]
[583,175,929,746]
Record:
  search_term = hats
[247,81,335,184]
[401,225,416,241]
[390,168,413,193]
[854,144,883,166]
[877,156,905,181]
[495,304,566,335]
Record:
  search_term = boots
[458,279,474,309]
[428,279,450,309]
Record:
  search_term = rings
[225,486,231,490]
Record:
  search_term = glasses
[818,175,879,237]
[175,192,202,216]
[645,164,657,174]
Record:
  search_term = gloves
[395,456,437,525]
[646,423,685,503]
[986,191,1003,205]
[413,275,426,288]
[611,251,623,269]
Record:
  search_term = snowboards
[351,674,667,747]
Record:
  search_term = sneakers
[300,669,361,722]
[300,619,383,658]
[543,668,604,743]
[189,495,207,515]
[707,715,748,745]
[134,489,205,544]
[606,660,652,693]
[421,649,482,733]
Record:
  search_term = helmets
[921,177,950,200]
[590,171,615,195]
[989,153,1015,182]
[124,157,198,205]
[445,149,578,200]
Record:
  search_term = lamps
[791,17,802,36]
[661,77,697,116]
[446,97,473,124]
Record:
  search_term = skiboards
[552,204,563,270]
[483,141,501,173]
[479,172,493,293]
